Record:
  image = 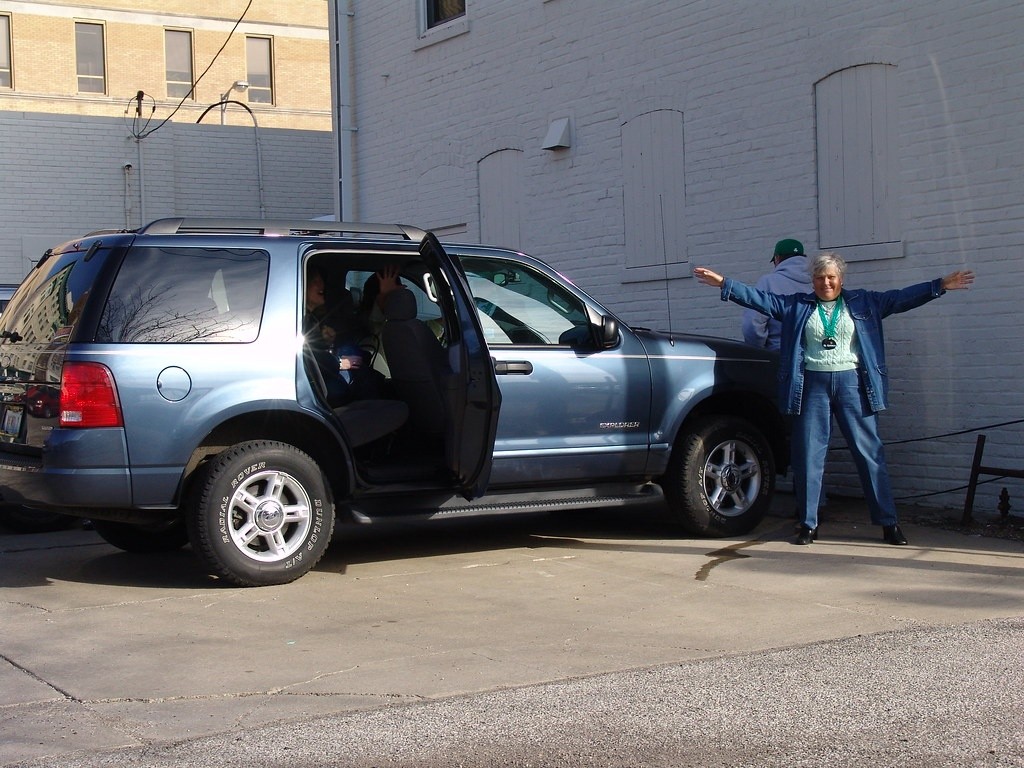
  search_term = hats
[769,239,807,263]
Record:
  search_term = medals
[822,338,836,349]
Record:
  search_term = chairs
[380,290,448,383]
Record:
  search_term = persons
[742,239,828,525]
[693,253,975,545]
[304,262,407,463]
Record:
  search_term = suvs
[0,216,791,587]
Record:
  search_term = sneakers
[797,526,817,544]
[883,525,907,544]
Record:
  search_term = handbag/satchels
[347,333,386,398]
